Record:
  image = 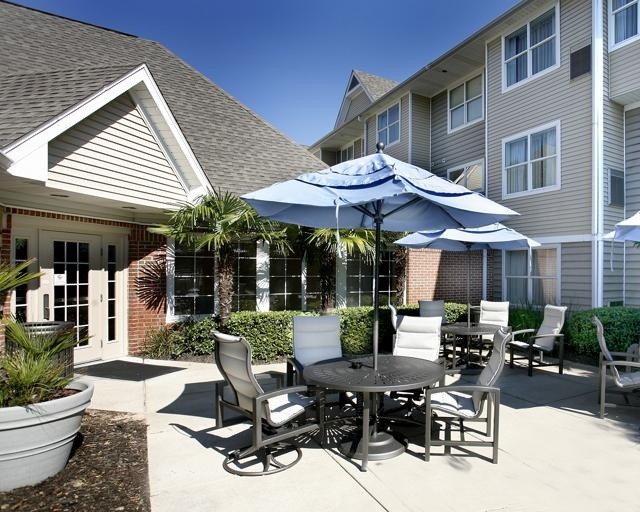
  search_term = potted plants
[0,309,95,496]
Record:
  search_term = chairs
[591,315,640,421]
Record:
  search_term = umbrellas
[597,210,640,245]
[239,141,521,437]
[393,223,541,364]
